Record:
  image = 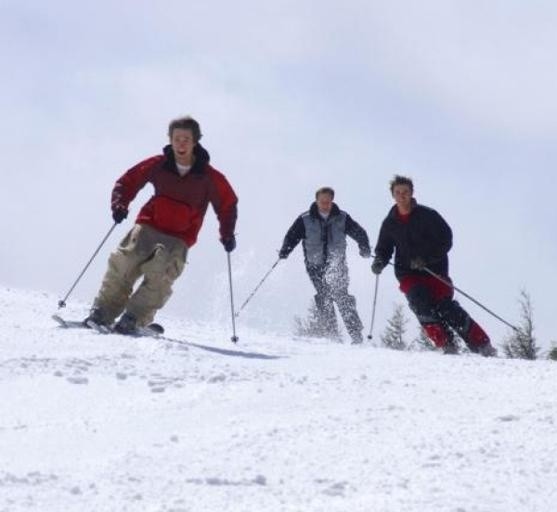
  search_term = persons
[369,174,492,356]
[82,119,239,334]
[278,185,371,341]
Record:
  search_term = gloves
[112,207,129,224]
[409,258,430,271]
[278,249,291,259]
[371,257,385,274]
[360,246,371,259]
[220,236,237,252]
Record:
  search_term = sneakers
[116,312,140,334]
[442,339,497,356]
[84,305,112,329]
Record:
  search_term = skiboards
[54,315,281,360]
